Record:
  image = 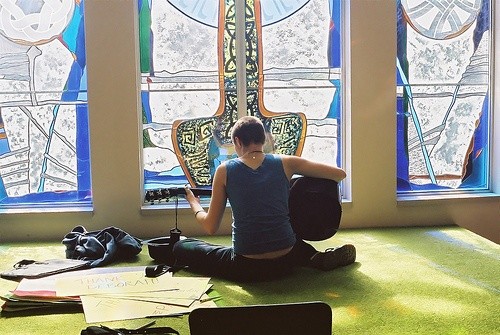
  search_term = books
[0,259,89,279]
[0,292,82,311]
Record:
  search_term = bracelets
[194,210,206,218]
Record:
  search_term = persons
[172,116,356,283]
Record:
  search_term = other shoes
[311,244,356,271]
